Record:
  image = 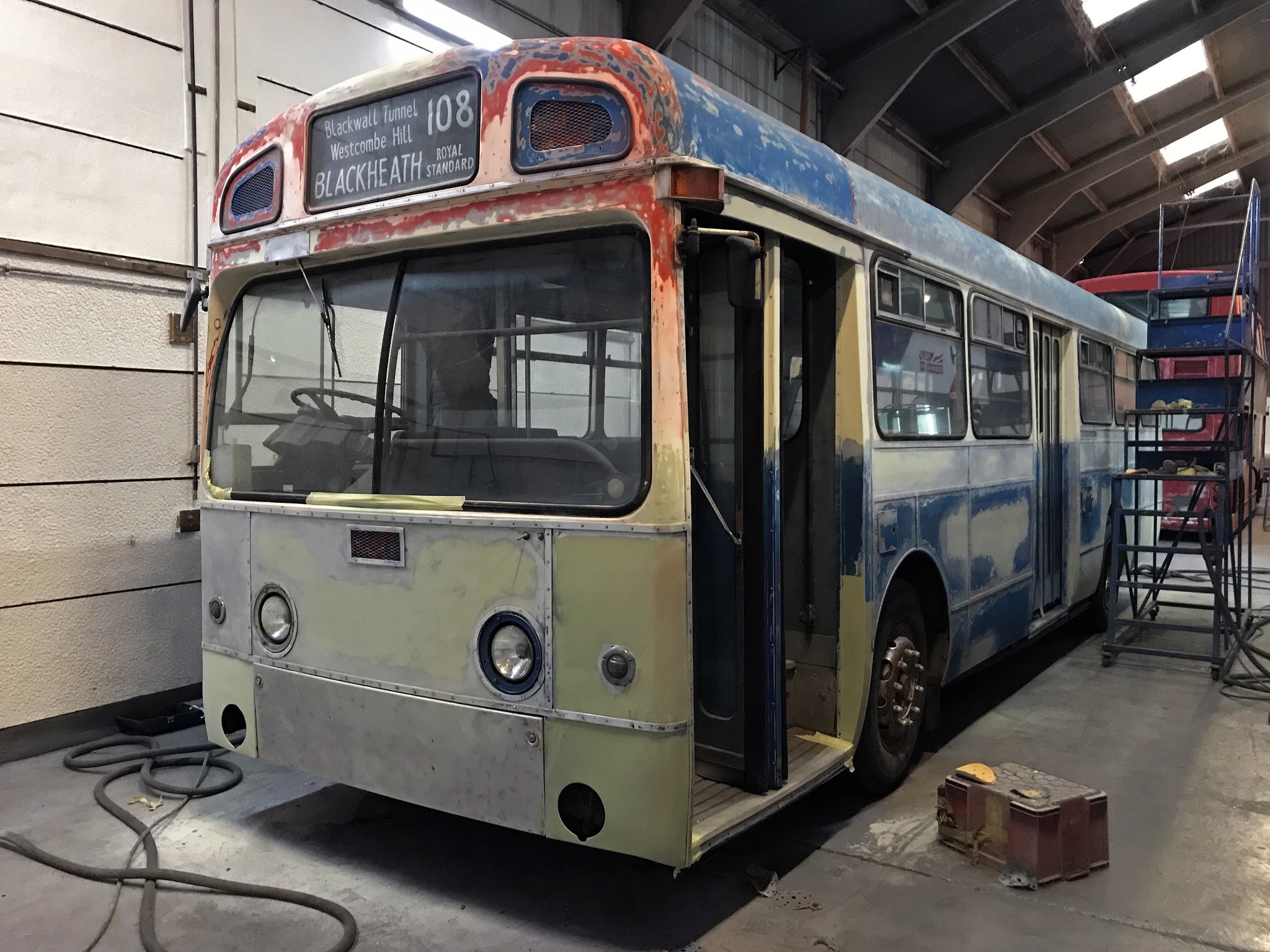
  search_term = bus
[168,33,1150,856]
[1074,270,1270,545]
